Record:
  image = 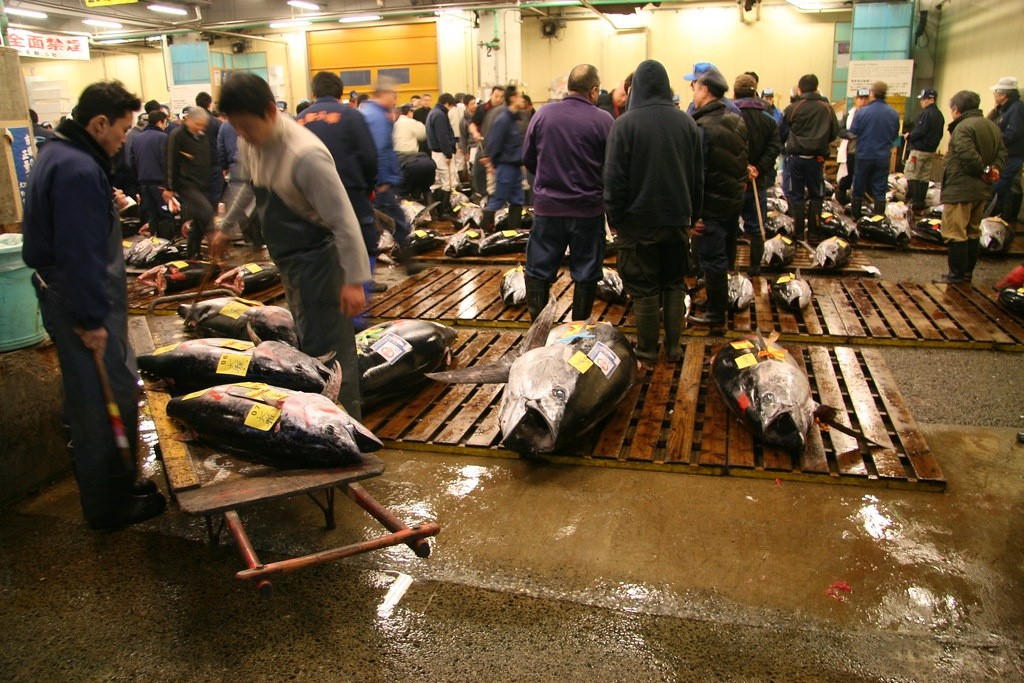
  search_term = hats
[762,88,774,98]
[683,62,719,81]
[857,88,871,99]
[916,89,937,100]
[988,76,1019,90]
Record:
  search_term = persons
[838,80,1024,284]
[20,80,167,530]
[288,71,538,290]
[209,73,372,424]
[519,58,840,362]
[30,91,265,265]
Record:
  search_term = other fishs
[213,261,282,296]
[124,231,204,270]
[725,270,756,314]
[710,314,900,452]
[423,290,661,458]
[136,318,386,467]
[176,295,301,350]
[592,265,633,306]
[135,258,223,296]
[375,187,537,267]
[499,260,530,306]
[656,282,715,329]
[770,265,813,311]
[355,316,460,401]
[764,168,1018,255]
[763,233,797,266]
[795,235,852,268]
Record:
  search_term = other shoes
[370,280,387,291]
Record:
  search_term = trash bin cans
[0,232,45,349]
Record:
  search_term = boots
[150,222,157,238]
[807,199,822,238]
[524,275,550,324]
[507,204,523,231]
[942,238,981,282]
[851,197,862,221]
[686,274,728,326]
[907,178,929,213]
[435,189,451,219]
[572,280,597,320]
[662,289,683,362]
[632,293,661,363]
[483,209,495,235]
[874,201,886,217]
[61,423,167,531]
[933,240,968,284]
[788,200,805,241]
[159,220,175,242]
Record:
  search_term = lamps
[147,1,189,16]
[80,16,122,29]
[287,0,320,11]
[434,7,464,16]
[338,15,383,24]
[3,4,49,20]
[270,20,313,29]
